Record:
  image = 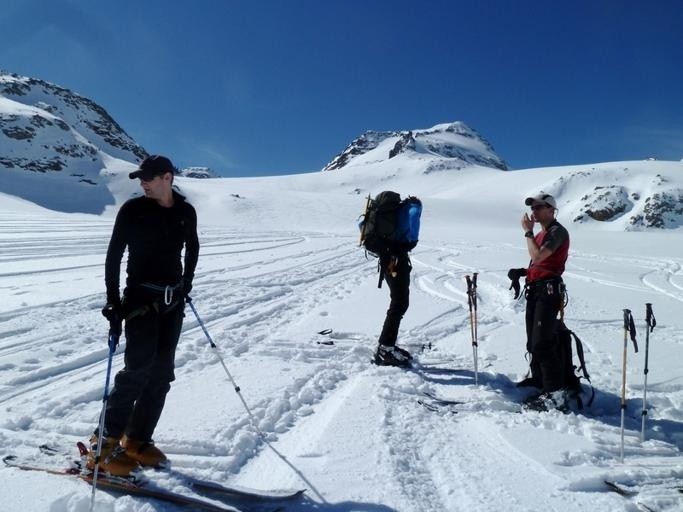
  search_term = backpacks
[540,319,591,400]
[357,191,409,256]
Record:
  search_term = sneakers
[375,344,412,368]
[87,432,166,477]
[524,387,571,414]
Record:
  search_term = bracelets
[524,231,533,238]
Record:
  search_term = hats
[129,155,174,179]
[525,193,556,209]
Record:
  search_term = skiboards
[371,349,467,374]
[417,391,589,415]
[2,444,307,512]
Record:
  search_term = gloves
[181,273,194,296]
[508,267,526,299]
[102,297,122,322]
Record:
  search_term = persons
[506,192,581,413]
[85,153,200,478]
[357,196,423,369]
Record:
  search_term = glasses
[531,205,548,211]
[138,172,156,182]
